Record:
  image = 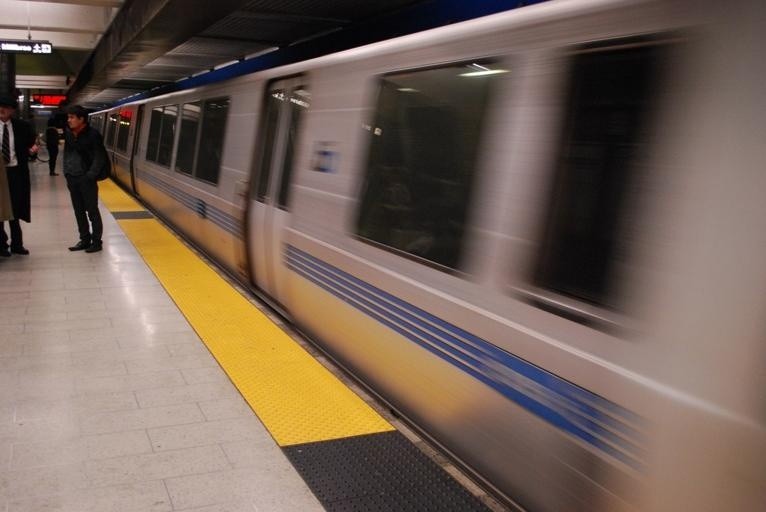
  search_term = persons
[46,119,59,176]
[0,94,41,256]
[62,105,110,253]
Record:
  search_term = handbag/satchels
[95,140,110,180]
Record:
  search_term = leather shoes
[69,241,91,251]
[11,248,28,254]
[86,244,101,252]
[0,250,11,257]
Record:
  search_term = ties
[2,124,10,163]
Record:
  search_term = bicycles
[26,133,50,163]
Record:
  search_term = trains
[64,1,765,510]
